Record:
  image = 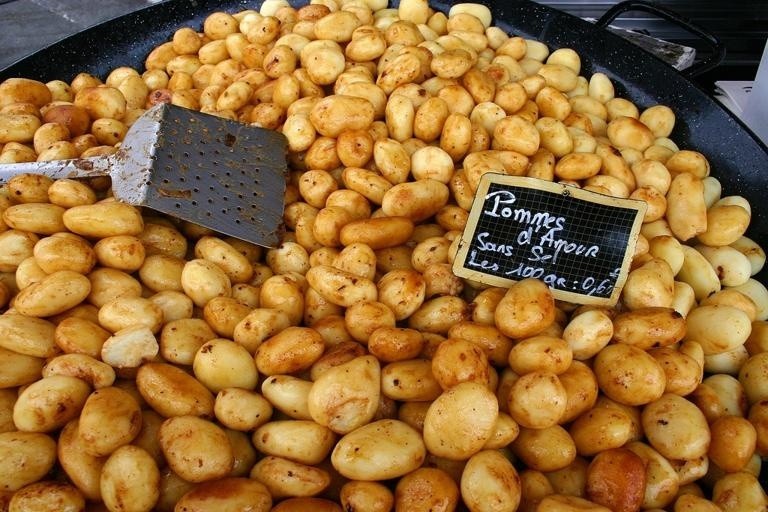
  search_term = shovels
[0,103,289,250]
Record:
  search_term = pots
[0,1,767,290]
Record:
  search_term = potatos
[0,1,768,512]
